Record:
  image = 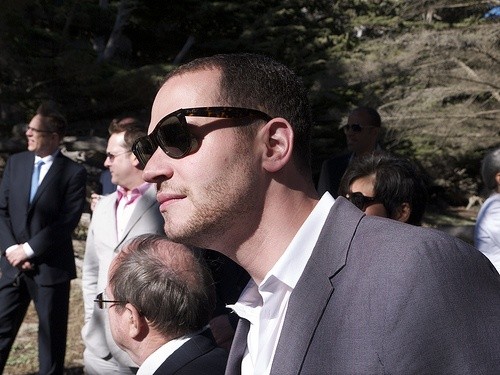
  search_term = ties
[29,160,45,204]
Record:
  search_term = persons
[1,102,87,374]
[95,234,230,374]
[80,121,166,374]
[132,52,499,375]
[90,115,144,215]
[317,105,383,201]
[472,149,499,277]
[339,148,426,228]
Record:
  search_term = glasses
[130,107,273,169]
[27,126,53,135]
[341,191,391,212]
[94,292,132,309]
[106,150,133,163]
